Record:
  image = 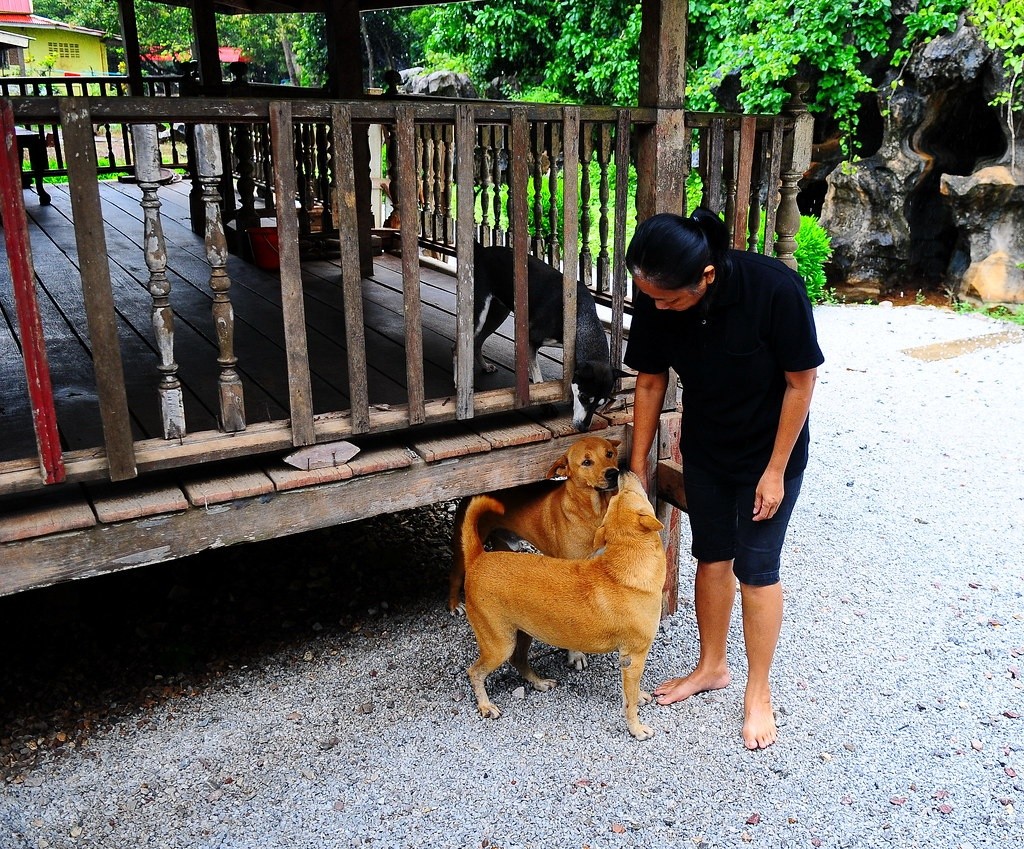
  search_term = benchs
[14,125,51,206]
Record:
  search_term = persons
[623,207,825,750]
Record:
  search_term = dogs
[451,236,638,432]
[457,436,668,742]
